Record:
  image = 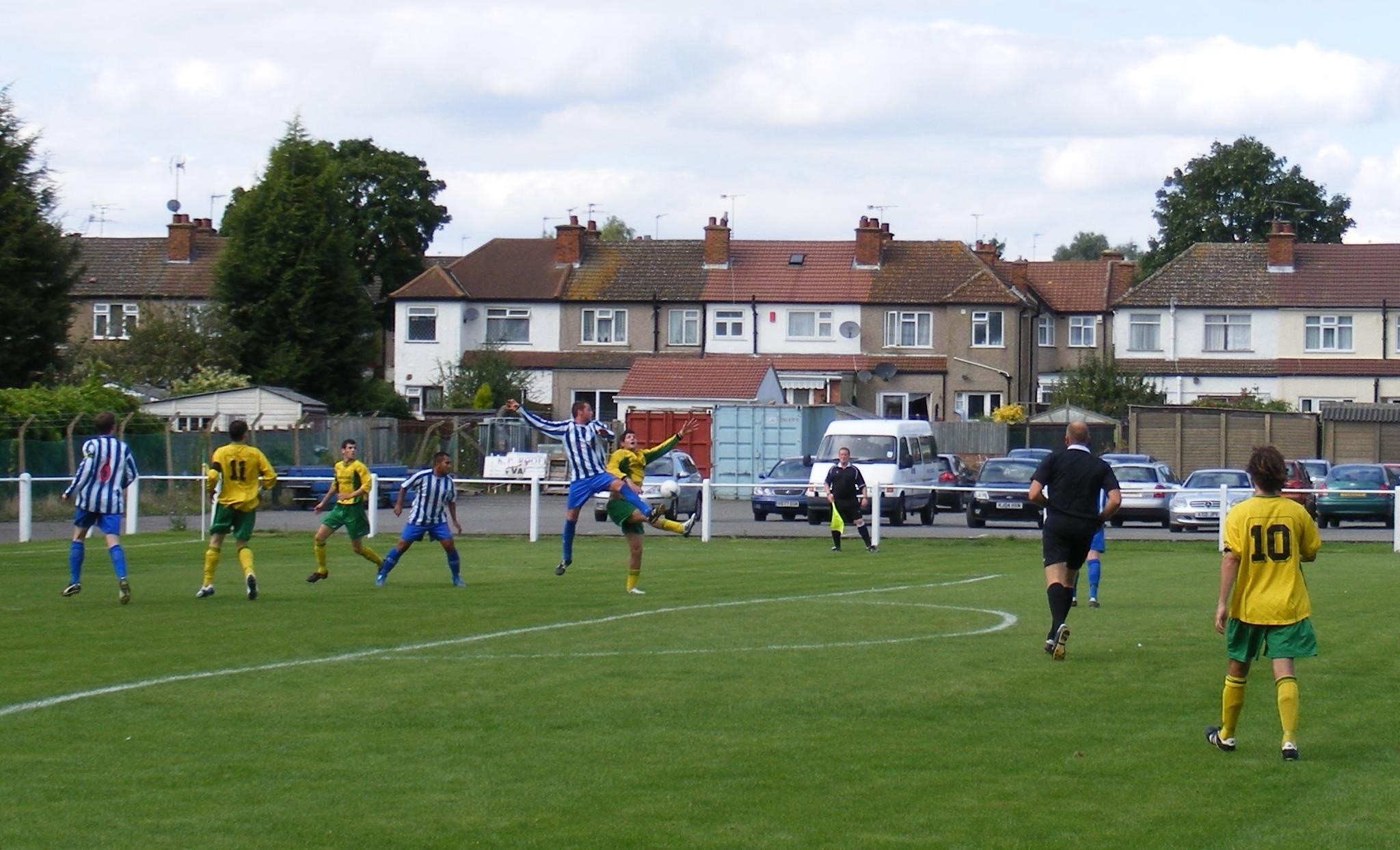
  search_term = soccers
[660,479,681,500]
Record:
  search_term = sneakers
[62,582,81,597]
[377,559,387,574]
[119,579,131,604]
[247,573,258,600]
[1205,726,1234,752]
[197,585,215,598]
[376,574,387,586]
[307,570,329,582]
[555,559,572,575]
[1281,742,1299,761]
[682,513,696,538]
[866,545,880,554]
[453,577,466,587]
[647,504,665,524]
[629,587,646,595]
[1045,625,1071,660]
[831,546,841,553]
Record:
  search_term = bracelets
[678,431,684,439]
[862,497,867,499]
[827,493,831,496]
[624,477,630,481]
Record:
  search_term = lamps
[1193,376,1199,384]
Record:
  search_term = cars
[592,448,704,522]
[1294,458,1334,490]
[751,454,818,523]
[1278,459,1317,524]
[935,453,976,514]
[1315,463,1400,529]
[1168,468,1256,534]
[1007,447,1054,458]
[1098,452,1157,465]
[1098,462,1185,529]
[966,457,1049,530]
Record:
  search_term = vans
[802,418,942,526]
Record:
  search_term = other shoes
[1072,598,1079,607]
[1088,597,1099,608]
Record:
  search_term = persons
[1068,488,1107,607]
[375,450,468,588]
[823,447,881,553]
[61,411,138,604]
[1205,442,1322,762]
[306,440,385,585]
[195,420,277,600]
[1028,422,1121,661]
[505,398,666,576]
[606,417,701,595]
